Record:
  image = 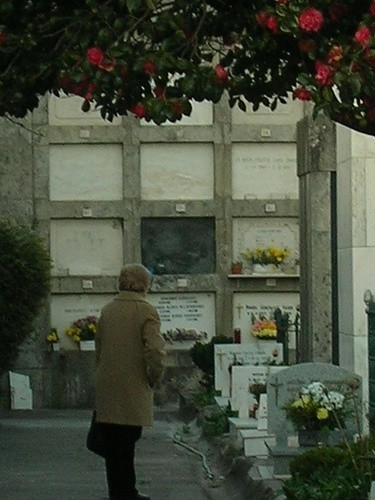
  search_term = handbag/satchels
[86,409,106,458]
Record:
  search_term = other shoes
[135,492,150,500]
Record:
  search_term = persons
[93,263,166,500]
